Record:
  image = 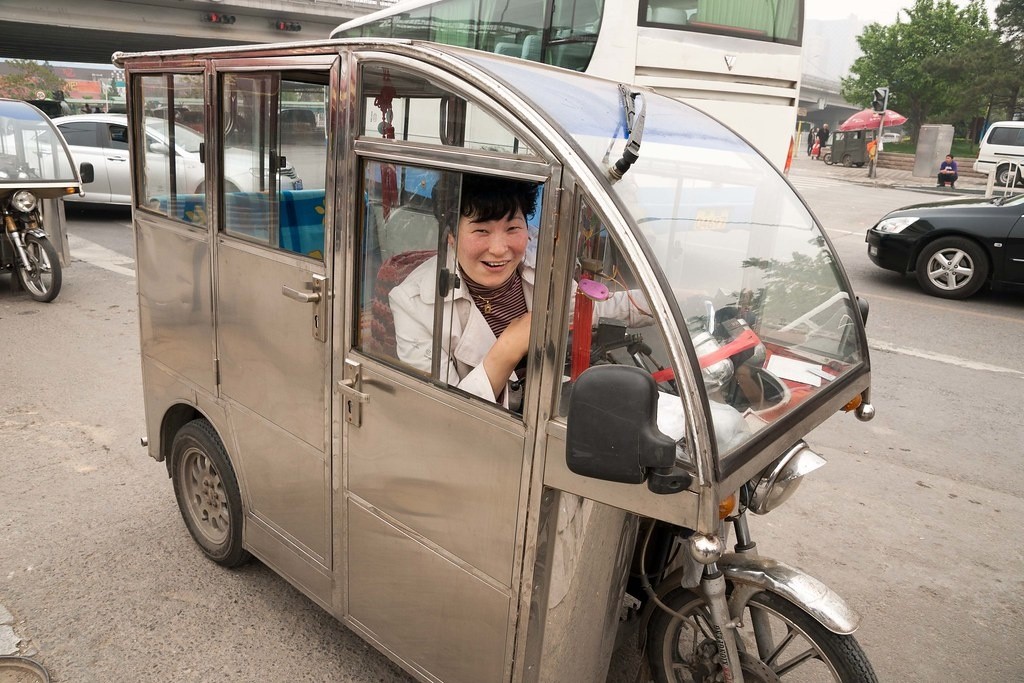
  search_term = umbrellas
[839,107,907,133]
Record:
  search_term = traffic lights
[206,13,236,24]
[275,21,301,31]
[870,87,888,115]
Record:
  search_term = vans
[279,109,317,132]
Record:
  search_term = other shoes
[807,152,812,156]
[951,184,956,190]
[937,184,944,188]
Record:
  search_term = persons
[807,124,830,156]
[938,154,958,189]
[388,149,711,410]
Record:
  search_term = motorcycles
[823,126,877,168]
[0,97,87,304]
[114,50,878,683]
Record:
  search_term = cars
[0,113,303,212]
[865,190,1023,299]
[176,112,206,133]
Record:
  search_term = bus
[324,2,806,232]
[972,120,1024,185]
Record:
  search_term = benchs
[149,187,386,312]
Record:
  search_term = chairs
[496,8,687,73]
